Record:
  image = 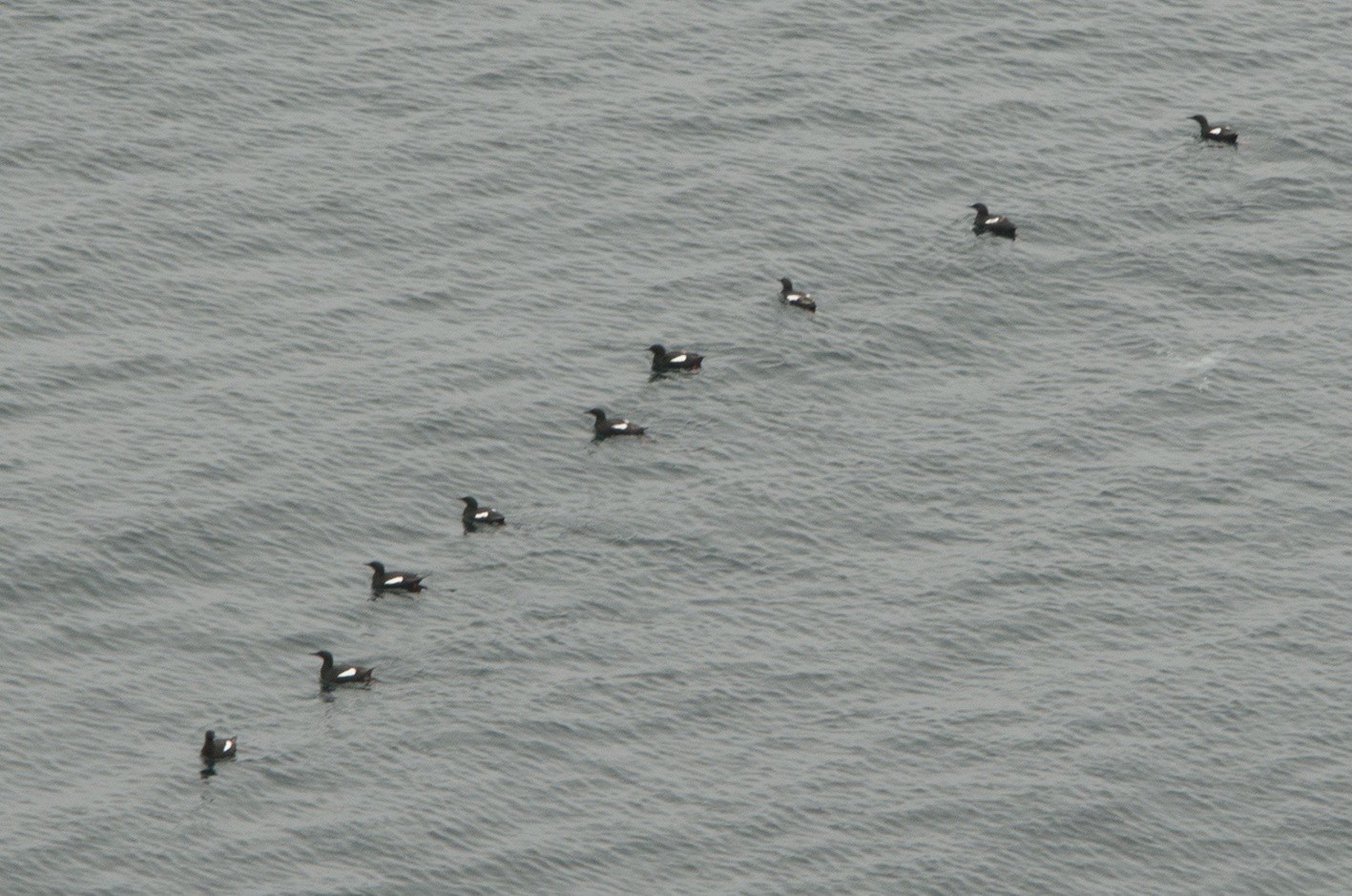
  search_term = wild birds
[966,204,1017,236]
[776,277,818,312]
[200,729,239,759]
[643,344,707,371]
[308,650,380,684]
[1185,113,1240,145]
[456,496,503,531]
[584,409,648,440]
[364,562,435,592]
